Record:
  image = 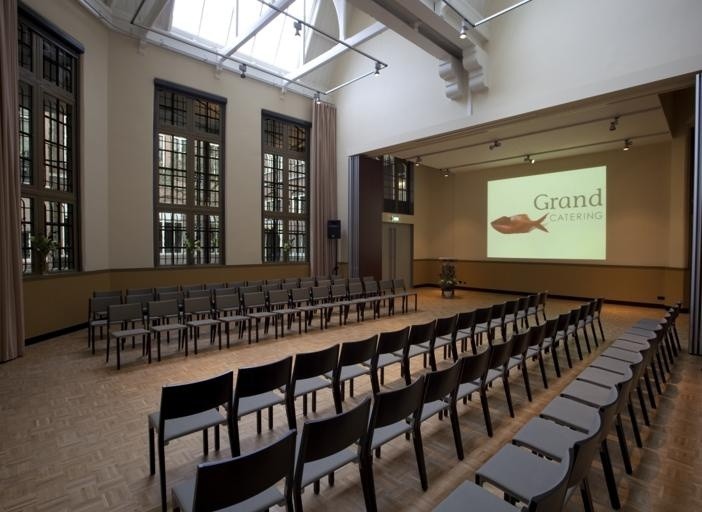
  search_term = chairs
[83,268,423,377]
[437,261,459,298]
[144,287,691,511]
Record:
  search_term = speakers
[327,219,341,239]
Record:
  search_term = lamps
[458,15,472,41]
[237,13,390,107]
[411,110,624,174]
[435,133,637,179]
